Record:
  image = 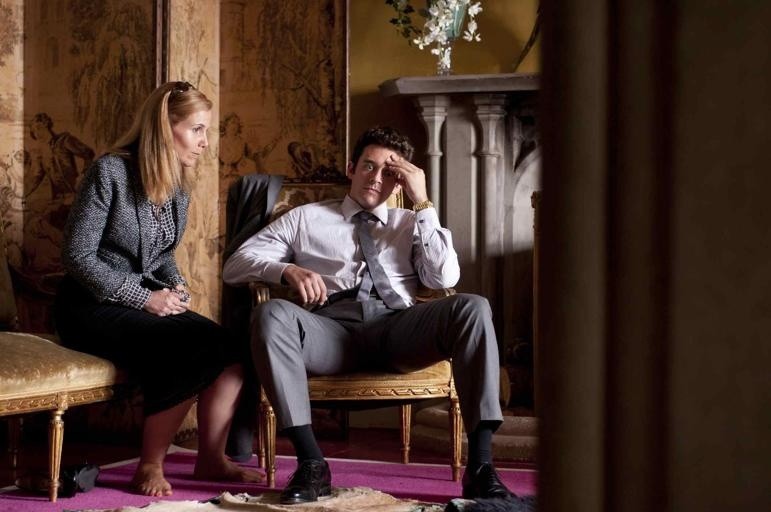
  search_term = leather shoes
[74,465,100,493]
[284,459,333,503]
[461,464,520,502]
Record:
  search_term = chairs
[8,256,67,335]
[245,178,465,487]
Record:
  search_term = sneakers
[14,464,77,496]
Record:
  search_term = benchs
[1,236,144,502]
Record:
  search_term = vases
[431,31,453,75]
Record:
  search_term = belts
[312,286,358,312]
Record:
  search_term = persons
[55,82,266,496]
[222,125,517,502]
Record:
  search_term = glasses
[168,81,190,101]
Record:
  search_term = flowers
[388,0,488,65]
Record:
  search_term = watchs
[411,200,433,210]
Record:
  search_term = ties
[353,210,409,312]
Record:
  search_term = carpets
[63,482,448,512]
[1,443,540,512]
